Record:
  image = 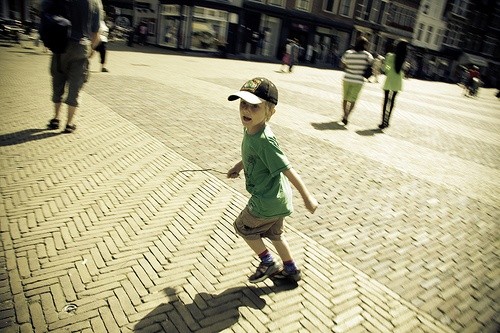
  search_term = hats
[228,77,278,104]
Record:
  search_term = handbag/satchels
[364,67,372,79]
[38,0,74,74]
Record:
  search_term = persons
[93,9,112,72]
[227,77,318,283]
[377,38,411,129]
[465,65,480,95]
[38,0,103,133]
[282,38,304,72]
[368,54,381,83]
[338,36,374,125]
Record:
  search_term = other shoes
[379,123,389,128]
[249,260,283,283]
[338,120,348,125]
[272,269,302,281]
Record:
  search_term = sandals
[64,124,76,133]
[50,118,60,129]
